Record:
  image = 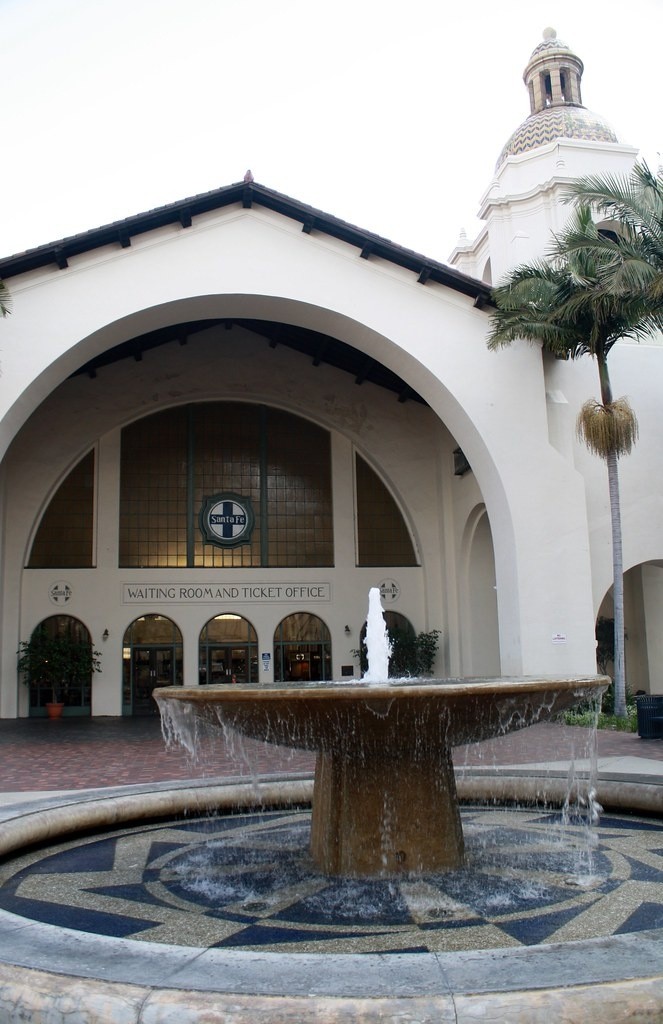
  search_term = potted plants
[16,620,103,721]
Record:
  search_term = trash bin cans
[633,694,663,739]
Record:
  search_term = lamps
[103,629,108,638]
[345,625,350,634]
